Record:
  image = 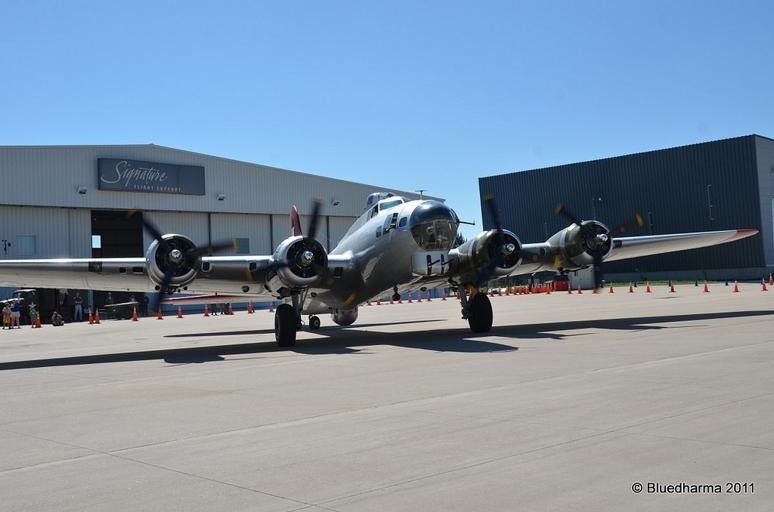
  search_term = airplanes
[0,188,761,347]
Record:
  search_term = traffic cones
[35,272,774,329]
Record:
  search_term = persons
[1,291,63,329]
[73,292,83,322]
[105,292,114,318]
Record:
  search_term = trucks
[0,288,58,325]
